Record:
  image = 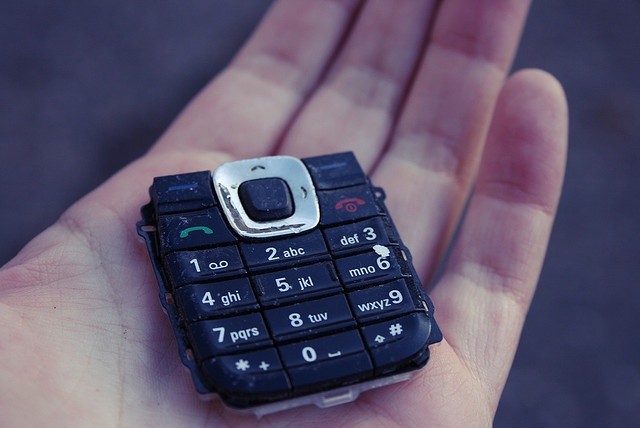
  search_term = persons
[0,0,568,428]
